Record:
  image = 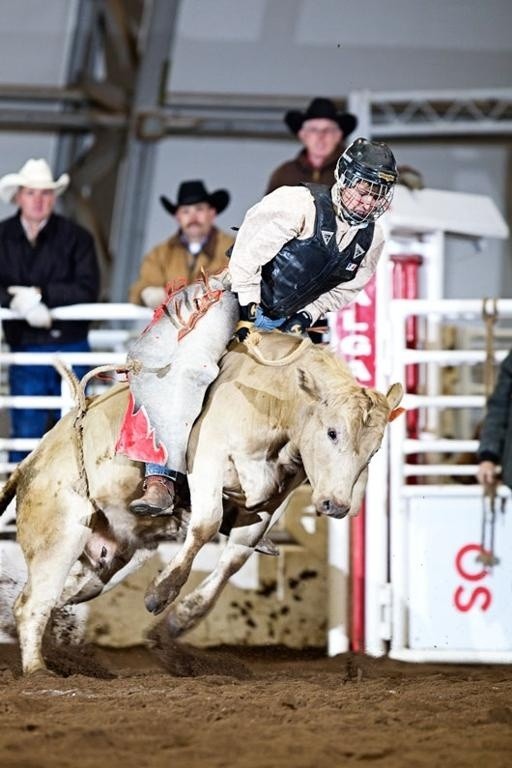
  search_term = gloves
[277,312,311,335]
[7,285,52,327]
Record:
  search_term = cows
[0,318,405,677]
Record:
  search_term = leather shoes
[129,476,174,518]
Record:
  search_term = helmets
[335,138,398,224]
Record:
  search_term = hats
[285,98,357,140]
[0,159,71,202]
[160,181,229,214]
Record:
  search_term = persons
[122,135,399,557]
[1,155,101,480]
[475,343,512,498]
[262,92,361,197]
[131,177,237,313]
